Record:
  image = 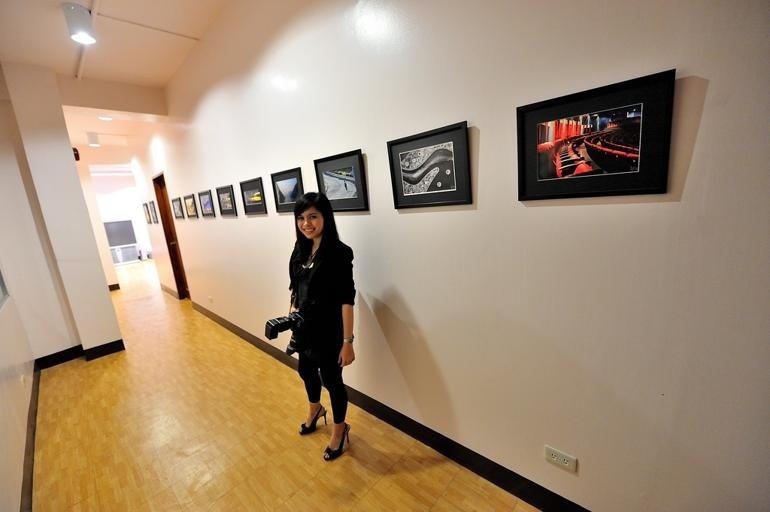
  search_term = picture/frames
[216,185,236,214]
[515,68,676,201]
[313,148,367,210]
[271,167,304,213]
[386,121,473,208]
[143,200,158,223]
[172,197,184,218]
[198,189,214,216]
[240,176,267,214]
[184,194,198,217]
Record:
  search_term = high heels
[299,405,327,435]
[323,422,350,461]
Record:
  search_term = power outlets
[542,445,579,473]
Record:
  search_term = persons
[289,192,355,461]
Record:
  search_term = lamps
[64,2,98,45]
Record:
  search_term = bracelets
[344,335,354,343]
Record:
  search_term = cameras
[265,311,306,357]
